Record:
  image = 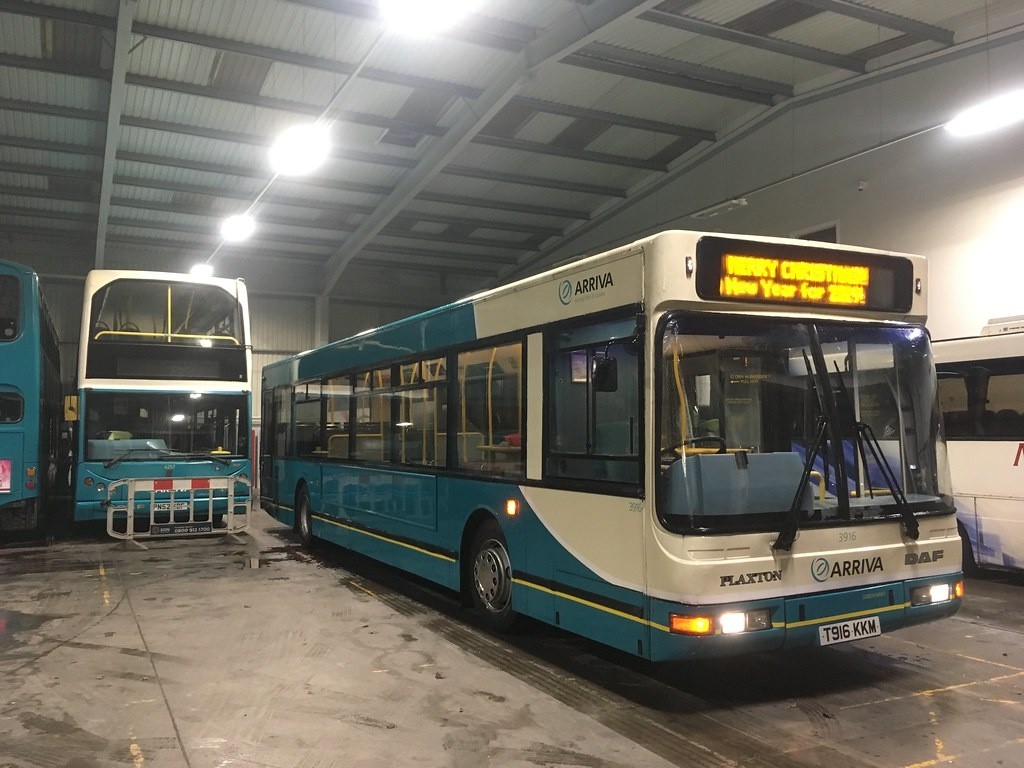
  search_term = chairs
[942,409,1024,440]
[290,421,639,482]
[91,321,111,340]
[119,322,139,341]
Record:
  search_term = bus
[0,259,62,531]
[260,229,962,663]
[70,270,253,526]
[695,315,1024,585]
